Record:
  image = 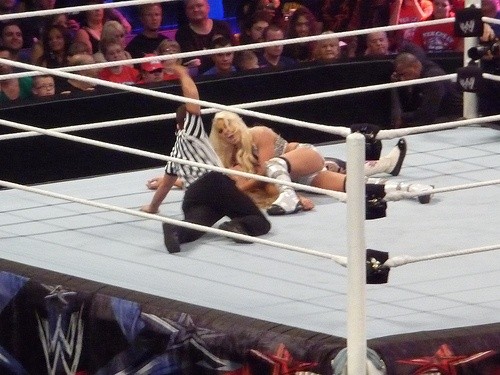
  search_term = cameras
[468,38,498,60]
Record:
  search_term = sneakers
[218,222,253,244]
[163,223,181,253]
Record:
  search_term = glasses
[37,83,55,89]
[163,48,179,55]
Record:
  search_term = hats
[141,53,164,71]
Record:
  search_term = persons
[147,111,435,216]
[139,62,271,254]
[0,0,500,129]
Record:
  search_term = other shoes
[390,138,406,176]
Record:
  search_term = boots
[365,177,435,203]
[263,160,304,215]
